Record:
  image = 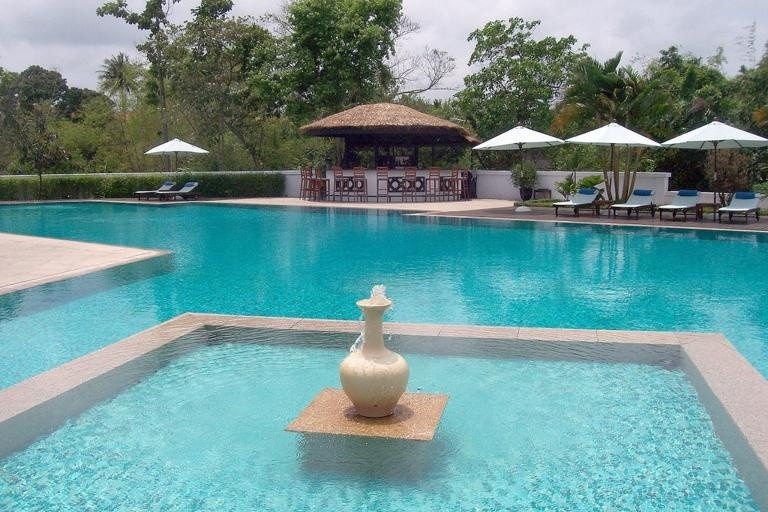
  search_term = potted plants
[510,160,536,201]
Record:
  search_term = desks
[695,202,719,221]
[593,199,613,215]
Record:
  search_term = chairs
[610,189,656,220]
[134,182,176,200]
[551,188,600,217]
[718,192,761,224]
[658,189,699,222]
[300,165,469,202]
[157,182,198,201]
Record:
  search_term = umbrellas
[658,121,767,221]
[143,138,209,200]
[469,126,563,213]
[565,121,662,216]
